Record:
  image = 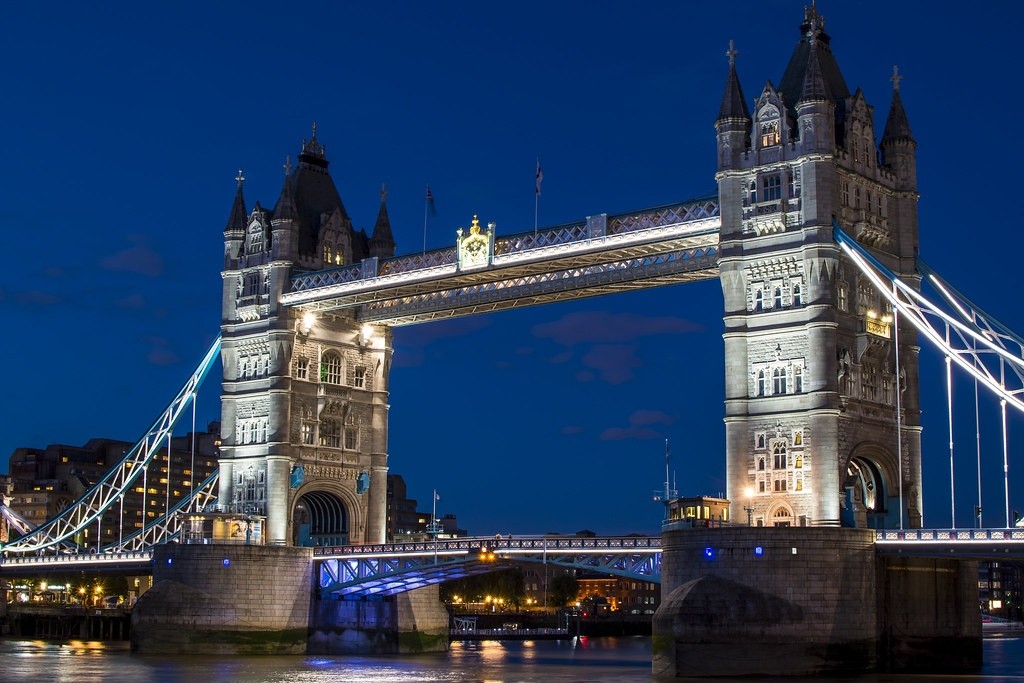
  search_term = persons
[247,527,252,540]
[495,533,512,539]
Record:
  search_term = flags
[436,494,440,500]
[535,161,544,196]
[427,189,433,200]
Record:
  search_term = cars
[981,616,993,623]
